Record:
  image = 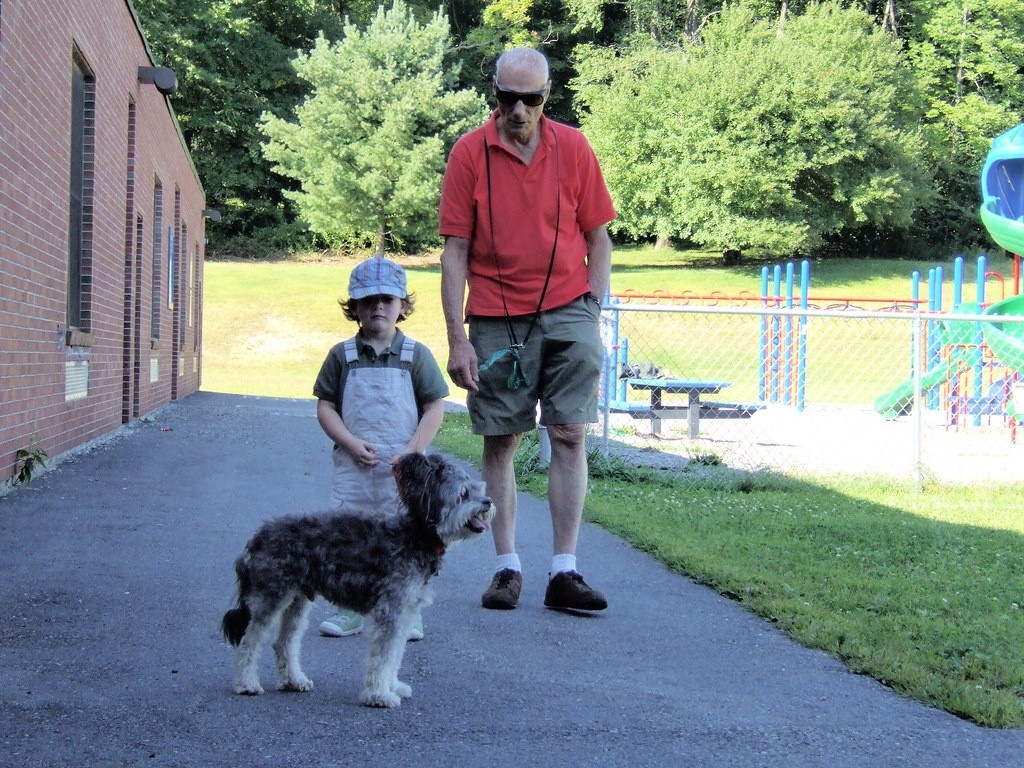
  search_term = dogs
[221,453,496,708]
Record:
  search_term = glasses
[494,81,547,107]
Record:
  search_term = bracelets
[589,294,602,312]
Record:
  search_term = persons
[439,48,619,609]
[313,257,449,641]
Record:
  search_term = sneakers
[319,610,363,636]
[482,568,522,607]
[407,615,423,639]
[544,570,608,610]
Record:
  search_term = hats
[348,258,407,298]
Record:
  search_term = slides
[873,123,1023,428]
